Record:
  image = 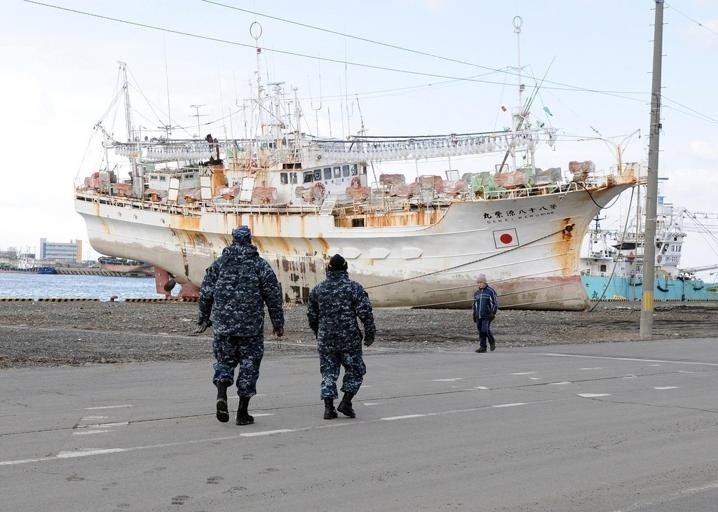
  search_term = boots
[338,391,355,418]
[236,396,253,424]
[324,398,337,419]
[217,385,229,422]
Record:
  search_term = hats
[232,226,250,244]
[477,273,486,284]
[328,254,347,271]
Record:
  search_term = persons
[471,272,499,353]
[304,252,376,419]
[192,224,287,426]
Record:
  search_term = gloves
[364,335,374,346]
[273,327,283,337]
[489,312,495,319]
[193,320,207,333]
[310,320,318,331]
[473,317,476,322]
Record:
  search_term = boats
[97,255,155,276]
[576,186,718,304]
[70,14,642,312]
[37,267,57,274]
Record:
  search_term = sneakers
[490,341,495,351]
[476,347,486,353]
